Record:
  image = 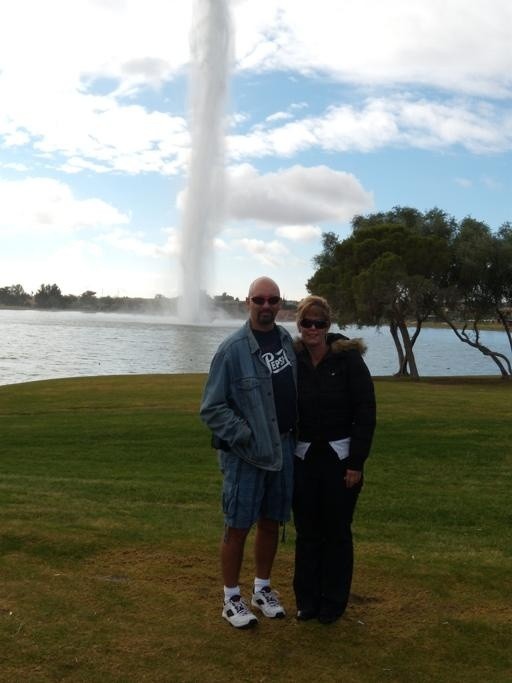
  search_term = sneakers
[222,595,258,628]
[251,586,286,618]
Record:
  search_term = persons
[199,277,301,630]
[290,295,376,624]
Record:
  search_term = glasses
[300,319,328,328]
[251,296,280,305]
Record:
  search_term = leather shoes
[296,608,339,624]
